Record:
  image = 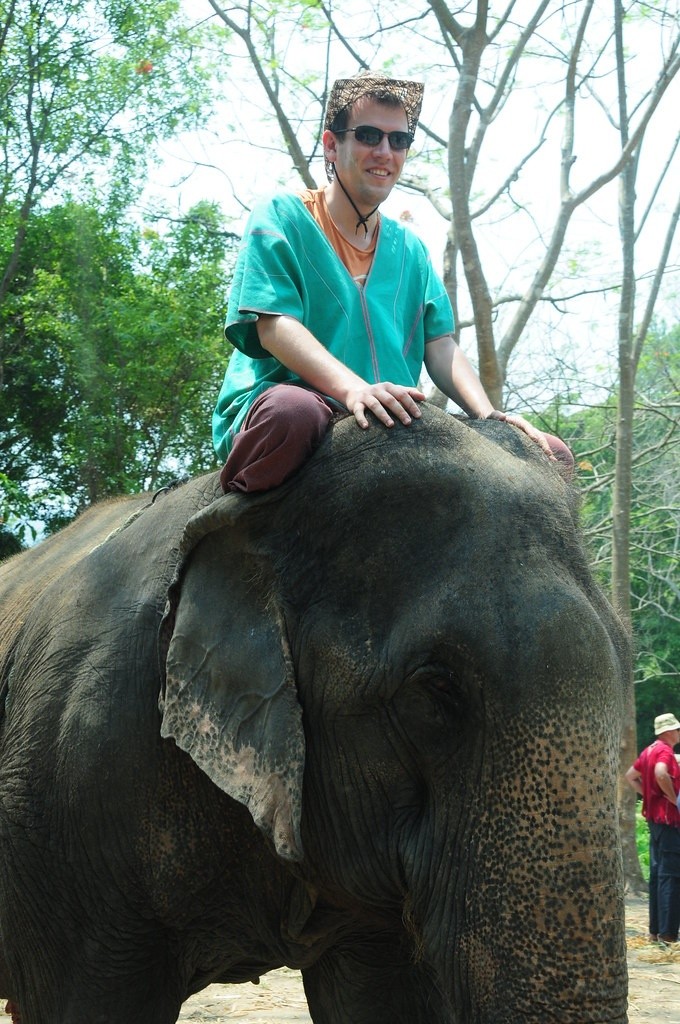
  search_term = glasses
[332,124,414,151]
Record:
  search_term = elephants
[0,397,640,1024]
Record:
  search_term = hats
[324,70,424,238]
[654,713,680,736]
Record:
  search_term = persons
[211,69,576,492]
[624,713,680,949]
